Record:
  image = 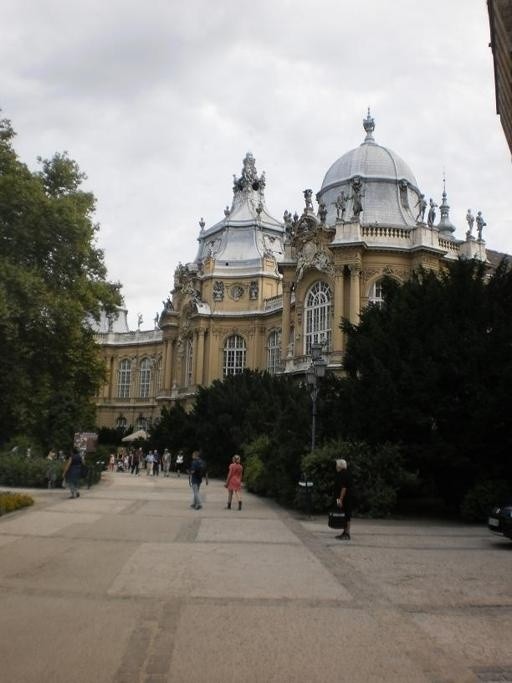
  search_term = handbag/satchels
[81,465,88,479]
[328,505,348,529]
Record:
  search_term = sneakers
[190,503,203,510]
[238,500,242,511]
[66,490,80,499]
[334,532,351,540]
[223,503,231,510]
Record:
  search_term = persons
[333,458,353,540]
[466,208,474,234]
[414,193,427,222]
[224,453,244,509]
[475,210,486,239]
[137,313,144,328]
[153,311,160,326]
[189,450,209,508]
[11,442,184,499]
[427,198,438,225]
[282,181,363,234]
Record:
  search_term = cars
[484,498,512,540]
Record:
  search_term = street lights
[304,332,330,453]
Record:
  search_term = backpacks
[195,460,206,479]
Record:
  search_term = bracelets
[340,498,343,500]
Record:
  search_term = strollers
[117,458,125,471]
